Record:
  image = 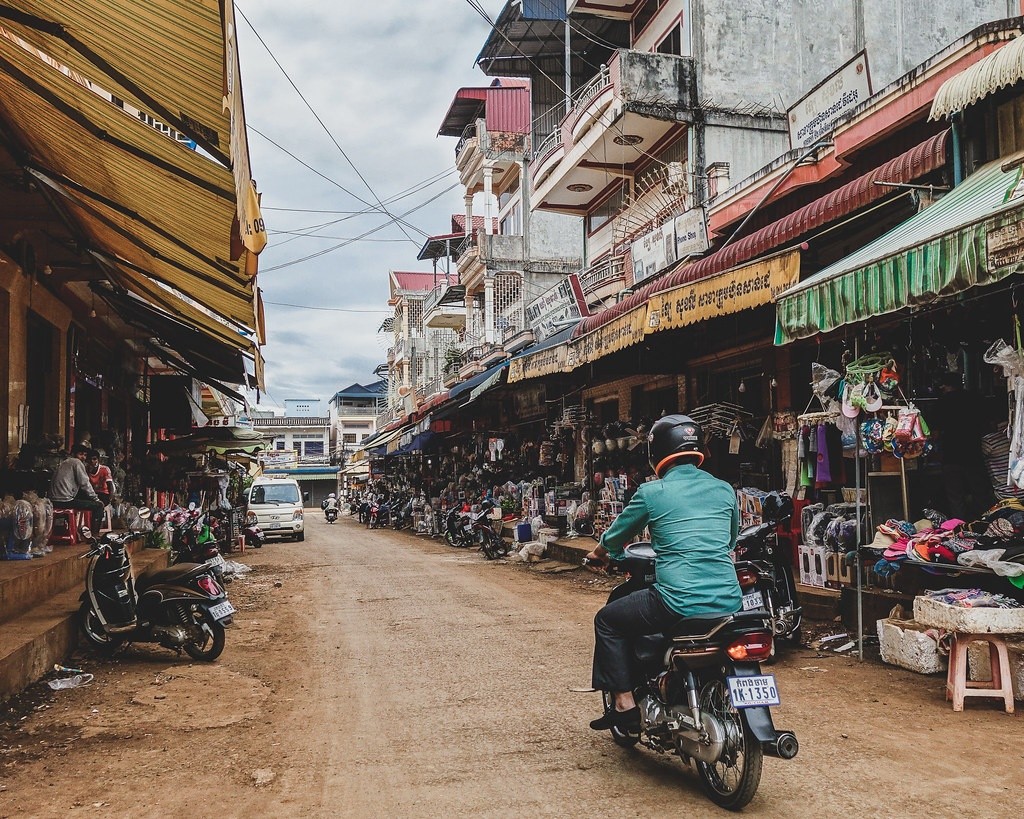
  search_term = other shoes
[589,702,642,730]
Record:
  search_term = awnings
[354,31,1024,452]
[0,0,265,416]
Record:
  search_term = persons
[82,449,120,509]
[980,412,1024,507]
[50,444,105,543]
[325,492,338,520]
[588,414,745,731]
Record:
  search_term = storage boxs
[528,484,582,517]
[877,594,1024,701]
[798,545,855,592]
[736,486,767,529]
[594,474,632,542]
[513,524,531,542]
[487,508,503,520]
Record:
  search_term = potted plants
[501,498,516,519]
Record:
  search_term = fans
[624,427,650,451]
[0,491,53,559]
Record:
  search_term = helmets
[762,490,793,518]
[646,415,704,476]
[328,493,336,499]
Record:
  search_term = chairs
[774,497,811,571]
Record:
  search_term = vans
[242,478,310,541]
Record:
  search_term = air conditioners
[662,161,683,194]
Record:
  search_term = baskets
[840,486,865,503]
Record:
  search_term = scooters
[366,497,383,528]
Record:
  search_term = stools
[99,506,112,532]
[946,630,1014,713]
[47,508,91,546]
[230,535,246,555]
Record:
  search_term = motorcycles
[571,516,800,812]
[77,506,237,664]
[470,501,507,561]
[444,503,476,548]
[731,497,805,666]
[222,500,267,548]
[166,502,233,592]
[323,501,339,524]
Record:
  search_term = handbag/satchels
[754,414,773,450]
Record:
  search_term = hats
[862,380,883,412]
[841,384,860,419]
[859,504,1024,564]
